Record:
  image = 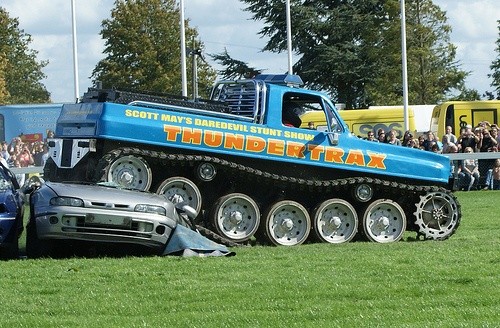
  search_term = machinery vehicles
[44,75,461,246]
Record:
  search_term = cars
[0,161,25,258]
[26,181,178,254]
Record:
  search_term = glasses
[368,134,374,136]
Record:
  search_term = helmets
[282,102,305,128]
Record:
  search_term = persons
[351,110,500,191]
[0,130,55,190]
[308,122,316,130]
[279,105,302,129]
